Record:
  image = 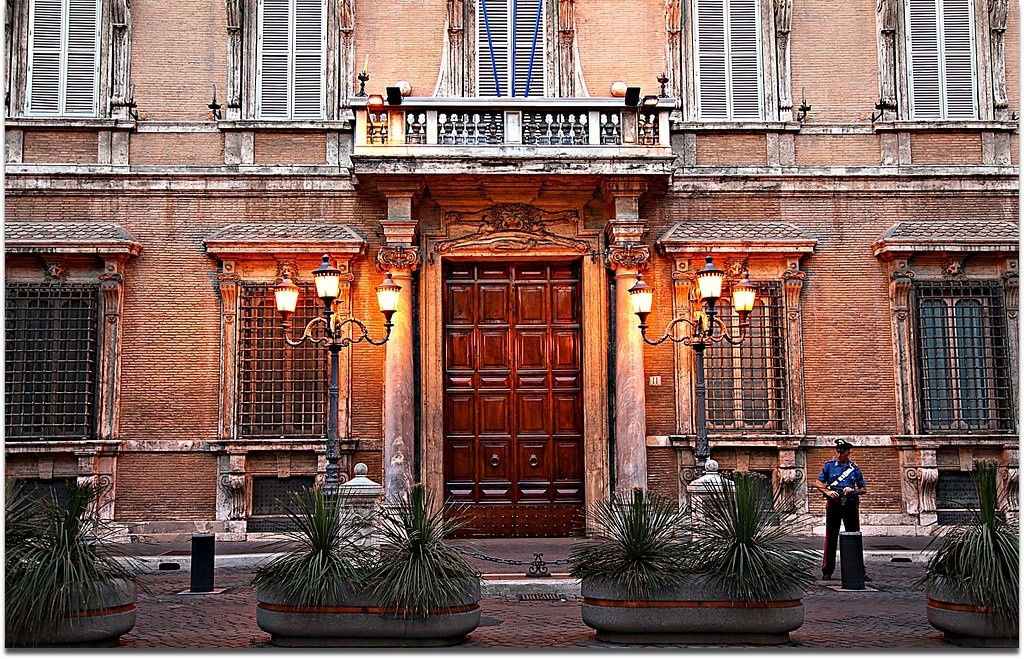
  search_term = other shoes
[864,573,873,582]
[822,574,831,580]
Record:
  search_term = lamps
[368,94,385,106]
[625,87,641,107]
[386,87,405,105]
[643,95,660,106]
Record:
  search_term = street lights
[627,255,757,480]
[272,254,401,553]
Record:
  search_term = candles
[662,59,665,75]
[364,54,369,73]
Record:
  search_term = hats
[835,439,853,452]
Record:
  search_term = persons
[815,439,873,581]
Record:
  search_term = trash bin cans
[190,534,215,592]
[839,531,866,590]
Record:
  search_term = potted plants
[911,456,1019,647]
[568,473,823,645]
[5,478,154,648]
[251,482,485,647]
